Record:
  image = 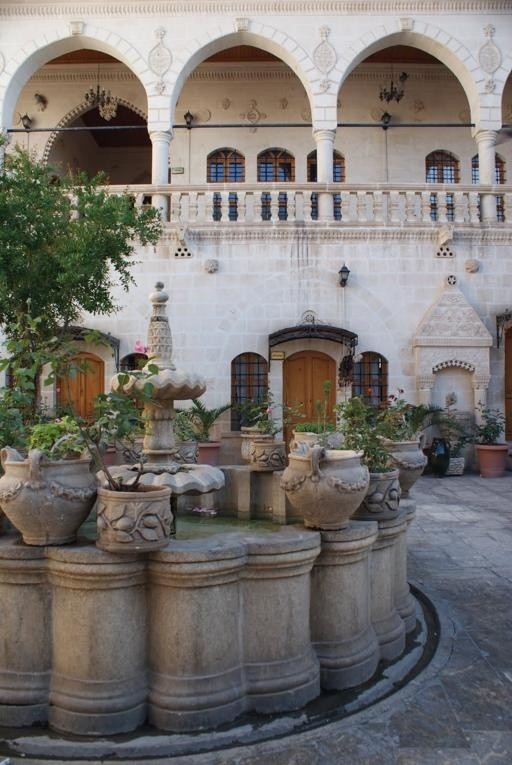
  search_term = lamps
[83,54,112,107]
[20,113,33,129]
[337,263,351,286]
[183,110,194,126]
[377,44,409,102]
[381,111,391,124]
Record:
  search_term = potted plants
[2,415,99,548]
[237,385,309,467]
[280,385,428,531]
[87,365,199,555]
[433,405,471,475]
[173,391,236,466]
[469,400,511,478]
